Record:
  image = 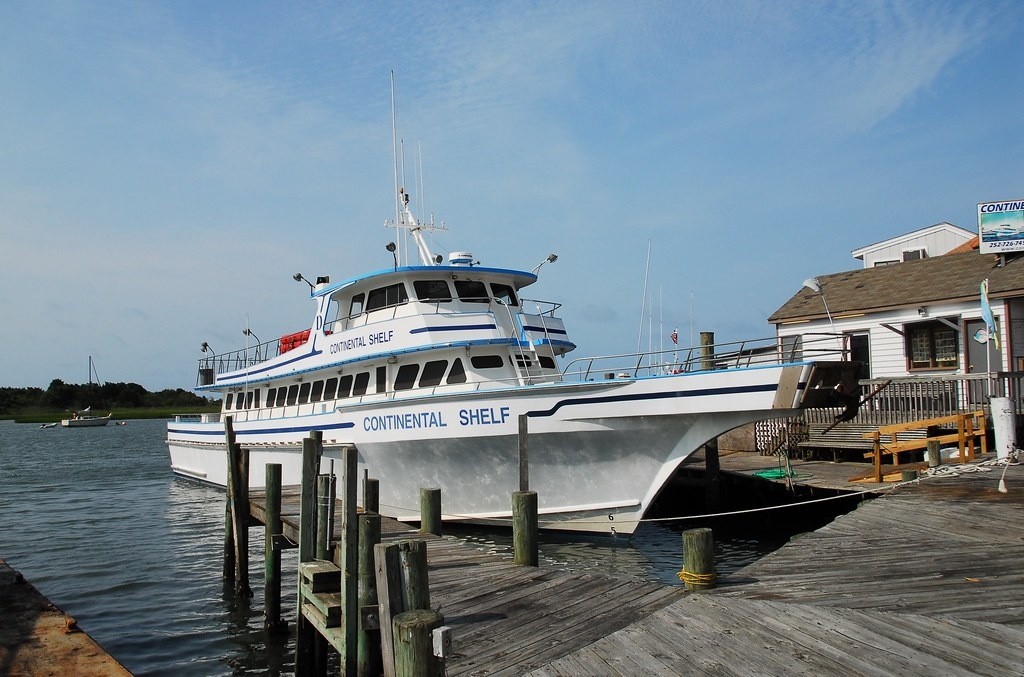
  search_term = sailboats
[61,355,114,427]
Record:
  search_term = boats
[115,421,126,426]
[164,68,869,541]
[39,423,58,428]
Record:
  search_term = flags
[979,278,1001,351]
[802,277,823,293]
[670,331,678,344]
